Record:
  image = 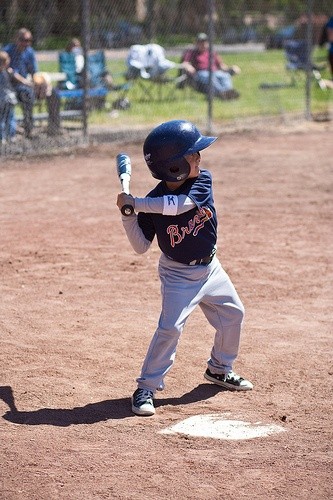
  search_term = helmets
[143,119,218,182]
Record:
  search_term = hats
[197,33,209,41]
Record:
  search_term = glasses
[18,39,33,43]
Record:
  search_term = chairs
[58,37,327,111]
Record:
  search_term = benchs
[15,89,110,132]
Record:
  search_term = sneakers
[131,389,156,416]
[204,368,254,391]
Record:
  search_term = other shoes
[46,127,68,135]
[1,139,7,144]
[318,78,327,90]
[222,90,240,98]
[26,132,40,138]
[10,137,15,144]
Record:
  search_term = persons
[182,32,239,100]
[5,30,65,139]
[116,120,254,417]
[1,50,16,138]
[59,37,116,90]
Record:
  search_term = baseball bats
[117,153,133,217]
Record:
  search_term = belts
[164,248,216,266]
